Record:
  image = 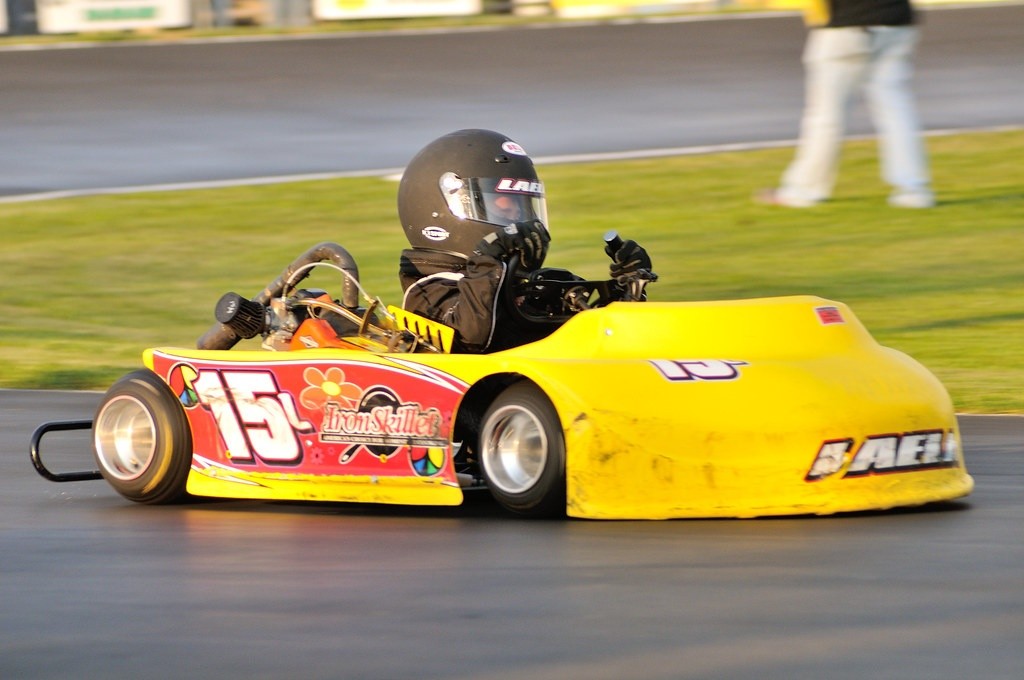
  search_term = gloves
[604,238,652,302]
[477,218,551,270]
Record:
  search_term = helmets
[396,128,550,260]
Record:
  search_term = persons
[752,0,936,208]
[398,129,652,354]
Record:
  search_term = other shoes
[891,183,934,209]
